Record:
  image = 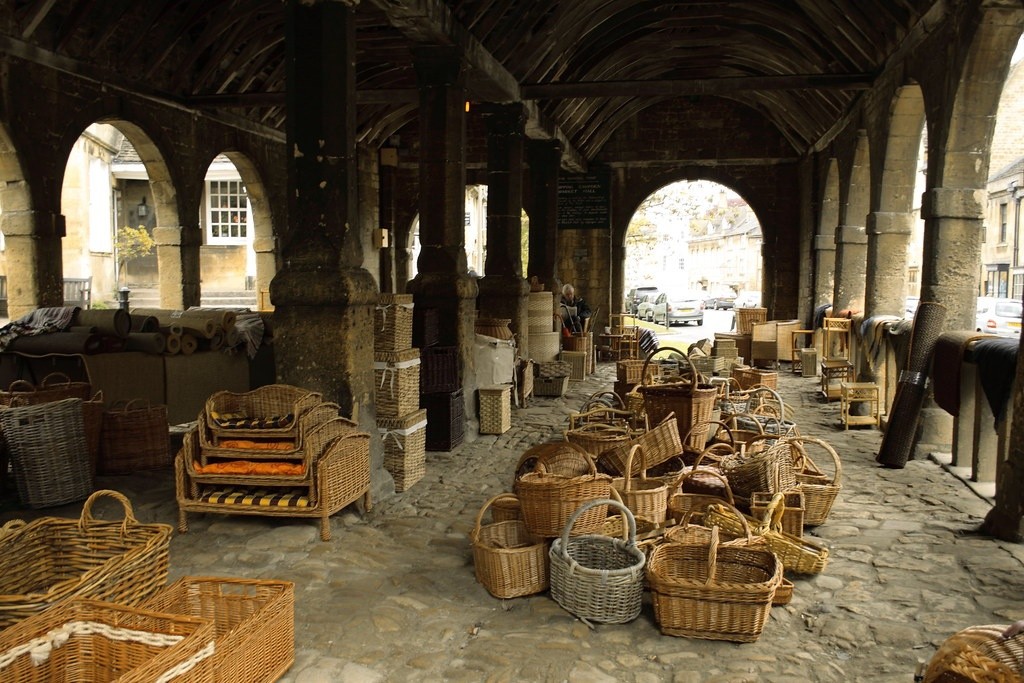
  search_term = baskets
[0,490,295,683]
[473,316,513,342]
[471,347,841,643]
[0,372,171,511]
[530,276,544,292]
[920,621,1024,683]
[735,307,767,335]
[563,335,587,352]
[373,292,427,493]
[610,327,633,335]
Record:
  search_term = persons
[561,284,592,332]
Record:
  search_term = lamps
[138,205,149,215]
[466,98,470,112]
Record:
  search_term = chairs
[749,318,801,370]
[587,306,600,332]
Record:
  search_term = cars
[633,292,662,322]
[702,284,737,310]
[652,290,704,328]
[733,290,761,312]
[903,296,920,322]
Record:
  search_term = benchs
[0,276,94,311]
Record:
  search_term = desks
[599,332,623,360]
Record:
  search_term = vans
[976,297,1023,340]
[625,287,657,315]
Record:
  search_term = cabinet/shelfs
[823,318,852,362]
[840,382,880,431]
[821,361,855,404]
[609,313,639,361]
